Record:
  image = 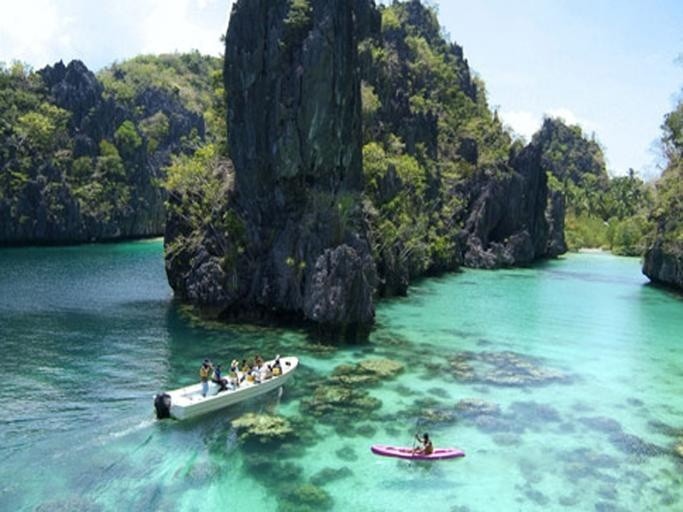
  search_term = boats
[153,356,299,422]
[371,445,464,459]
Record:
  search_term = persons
[199,354,282,396]
[415,432,432,455]
